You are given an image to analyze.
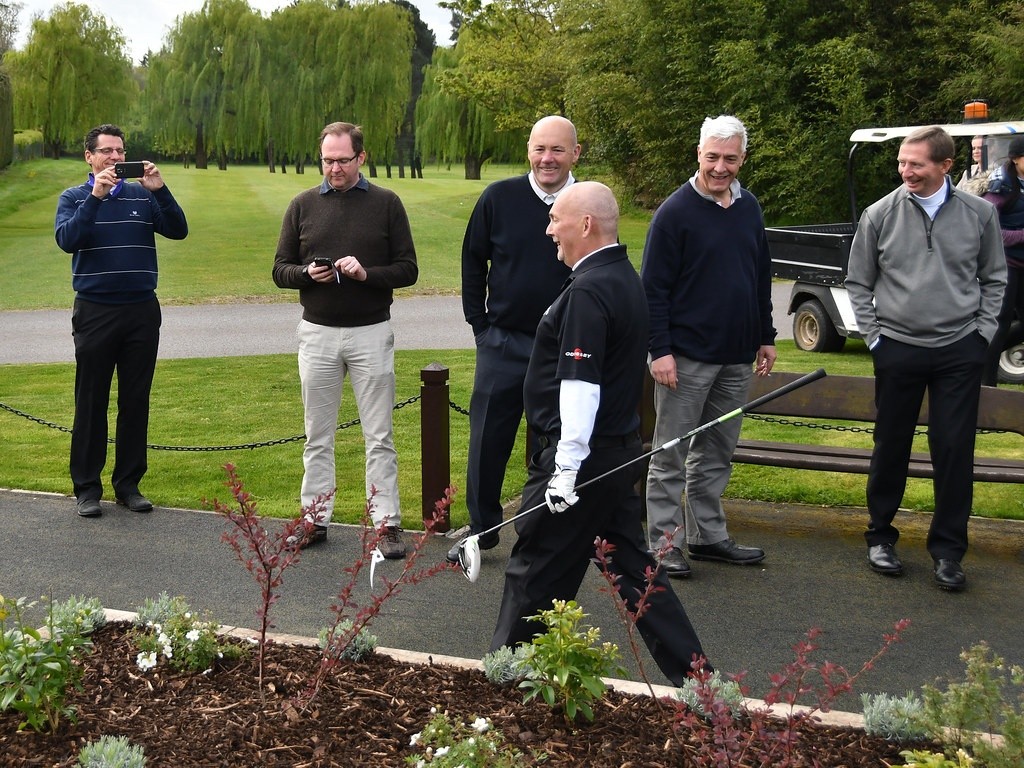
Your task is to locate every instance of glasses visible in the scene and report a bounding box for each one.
[320,152,359,167]
[92,147,127,155]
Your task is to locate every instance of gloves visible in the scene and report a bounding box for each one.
[545,464,579,514]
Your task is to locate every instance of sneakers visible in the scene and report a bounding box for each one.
[284,522,328,550]
[379,525,406,557]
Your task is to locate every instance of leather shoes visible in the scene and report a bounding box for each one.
[687,540,766,564]
[446,529,499,564]
[78,499,102,515]
[655,547,691,575]
[933,559,966,586]
[116,490,153,511]
[868,543,903,572]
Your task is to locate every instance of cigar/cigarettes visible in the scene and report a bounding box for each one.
[767,373,771,376]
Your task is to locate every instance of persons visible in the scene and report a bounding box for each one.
[492,182,719,689]
[846,127,1008,589]
[274,121,419,558]
[981,136,1024,387]
[443,115,620,562]
[955,134,1002,196]
[643,115,779,574]
[55,124,190,516]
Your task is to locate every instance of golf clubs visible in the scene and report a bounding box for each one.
[456,366,828,585]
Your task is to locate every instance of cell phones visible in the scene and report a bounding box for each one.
[314,258,333,273]
[114,162,144,179]
